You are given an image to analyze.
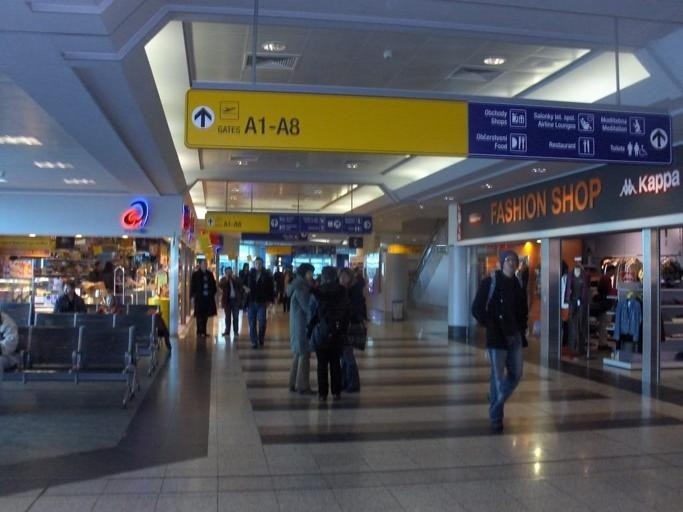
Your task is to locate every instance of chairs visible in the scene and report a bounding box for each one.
[0,303,157,405]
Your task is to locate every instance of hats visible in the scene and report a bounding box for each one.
[499,250,519,267]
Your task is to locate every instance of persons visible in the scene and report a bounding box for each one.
[471,251,529,433]
[54,261,118,372]
[0,312,20,373]
[219,257,368,401]
[190,260,217,337]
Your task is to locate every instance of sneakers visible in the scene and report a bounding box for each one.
[492,421,503,432]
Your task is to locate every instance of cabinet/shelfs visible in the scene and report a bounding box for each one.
[604,284,683,370]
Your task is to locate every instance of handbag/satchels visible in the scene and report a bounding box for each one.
[347,327,366,350]
[240,292,247,308]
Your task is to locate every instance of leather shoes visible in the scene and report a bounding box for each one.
[290,385,359,400]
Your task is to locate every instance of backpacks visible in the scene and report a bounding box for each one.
[312,287,351,342]
